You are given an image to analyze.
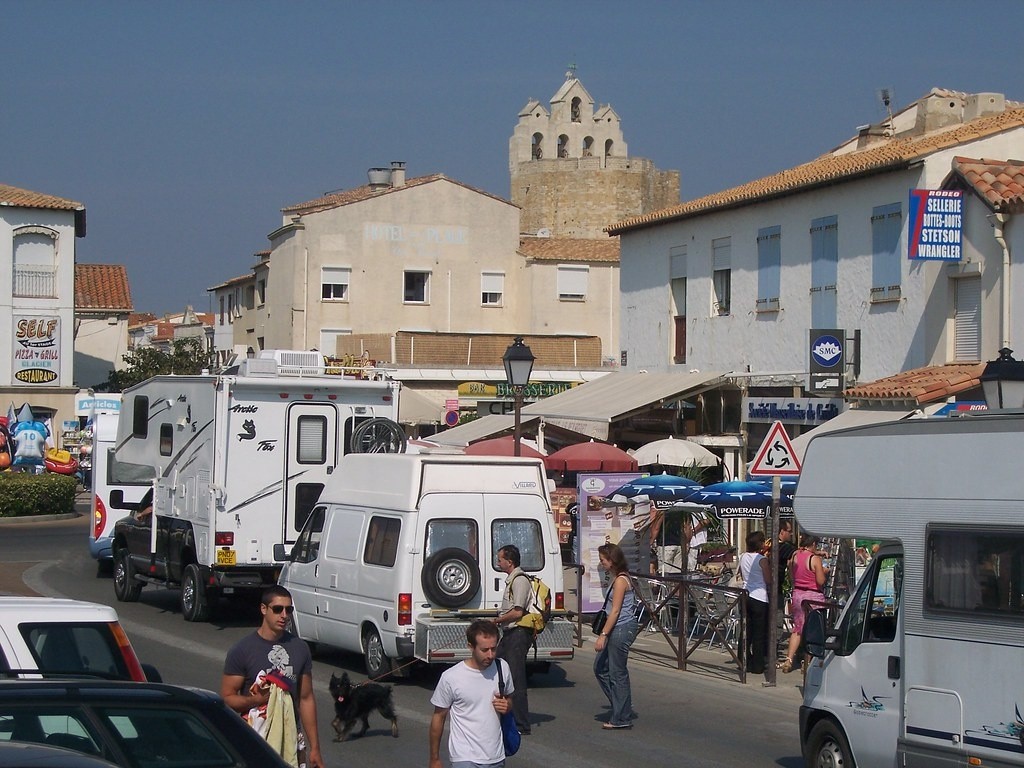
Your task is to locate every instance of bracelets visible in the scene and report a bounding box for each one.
[601,631,609,637]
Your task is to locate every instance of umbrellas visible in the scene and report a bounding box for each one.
[463,435,721,471]
[398,382,458,426]
[604,471,798,627]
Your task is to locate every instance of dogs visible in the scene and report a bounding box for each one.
[328,671,399,741]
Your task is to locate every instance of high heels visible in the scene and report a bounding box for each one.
[784,658,793,673]
[801,660,809,673]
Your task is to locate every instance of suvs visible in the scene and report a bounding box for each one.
[0,670,298,768]
[0,594,164,739]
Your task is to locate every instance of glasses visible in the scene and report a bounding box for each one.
[267,605,294,614]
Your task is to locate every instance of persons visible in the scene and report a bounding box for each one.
[220,586,324,768]
[137,506,152,520]
[649,545,658,575]
[859,544,879,567]
[687,508,711,571]
[79,447,89,473]
[593,544,638,729]
[739,516,832,674]
[493,544,533,735]
[429,620,514,768]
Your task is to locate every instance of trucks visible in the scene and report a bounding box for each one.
[90,413,155,574]
[108,350,400,620]
[788,411,1023,767]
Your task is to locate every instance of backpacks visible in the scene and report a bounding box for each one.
[510,572,552,633]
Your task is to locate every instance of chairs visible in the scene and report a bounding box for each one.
[631,573,798,653]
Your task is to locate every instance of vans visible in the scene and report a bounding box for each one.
[274,454,573,676]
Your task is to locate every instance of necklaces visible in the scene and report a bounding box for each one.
[799,548,814,553]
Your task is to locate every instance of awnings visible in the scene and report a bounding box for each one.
[747,407,919,476]
[422,414,540,446]
[507,370,733,440]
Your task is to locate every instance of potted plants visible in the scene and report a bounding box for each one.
[697,540,735,564]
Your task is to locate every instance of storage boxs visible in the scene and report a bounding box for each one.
[412,618,575,664]
[549,488,577,544]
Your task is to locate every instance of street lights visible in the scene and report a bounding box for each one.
[499,335,535,458]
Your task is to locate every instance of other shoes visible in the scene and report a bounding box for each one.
[602,721,632,730]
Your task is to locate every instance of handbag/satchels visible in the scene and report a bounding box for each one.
[593,610,607,634]
[495,658,522,756]
